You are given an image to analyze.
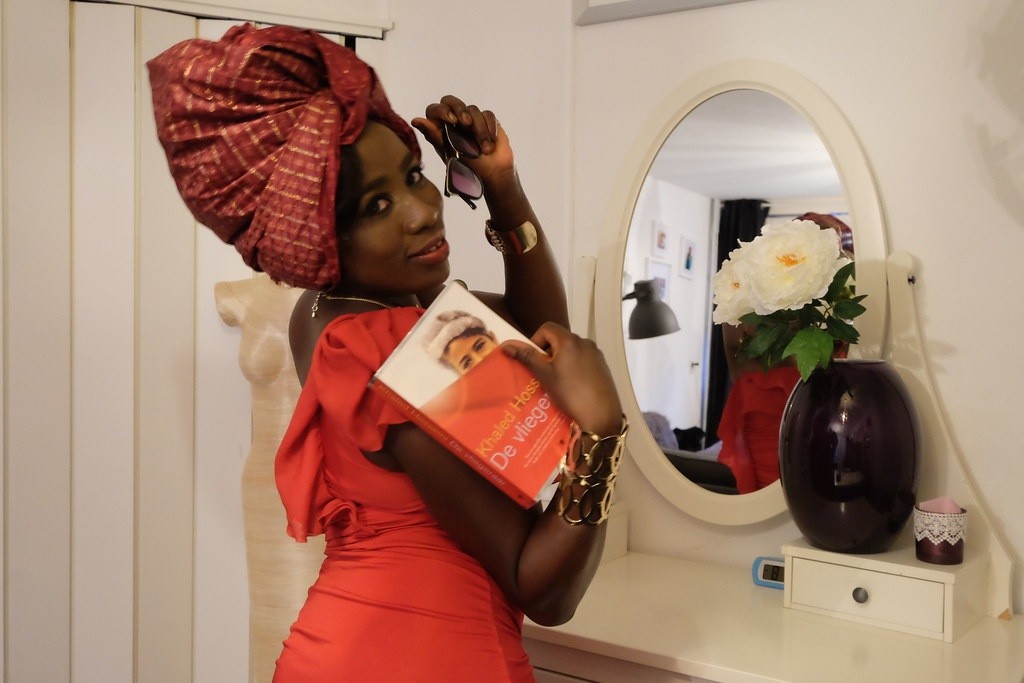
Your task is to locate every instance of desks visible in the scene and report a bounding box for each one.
[523,550,1024,683]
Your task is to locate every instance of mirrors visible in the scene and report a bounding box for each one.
[593,60,887,526]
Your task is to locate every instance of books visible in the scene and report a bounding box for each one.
[368,280,574,509]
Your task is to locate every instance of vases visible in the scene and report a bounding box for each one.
[779,358,915,552]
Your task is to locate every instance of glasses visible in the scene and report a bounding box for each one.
[442,118,484,210]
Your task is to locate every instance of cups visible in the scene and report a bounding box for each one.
[913,506,968,566]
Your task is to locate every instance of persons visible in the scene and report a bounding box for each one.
[421,310,500,374]
[214,273,329,682]
[144,19,631,683]
[715,212,853,495]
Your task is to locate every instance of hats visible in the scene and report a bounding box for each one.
[420,310,485,359]
[146,22,423,294]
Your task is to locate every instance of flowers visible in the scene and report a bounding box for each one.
[737,219,868,384]
[712,249,798,374]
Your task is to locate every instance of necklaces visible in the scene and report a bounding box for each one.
[310,290,421,321]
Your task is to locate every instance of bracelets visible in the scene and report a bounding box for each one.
[485,218,538,256]
[546,411,629,523]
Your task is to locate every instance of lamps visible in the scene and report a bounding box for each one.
[622,279,680,339]
